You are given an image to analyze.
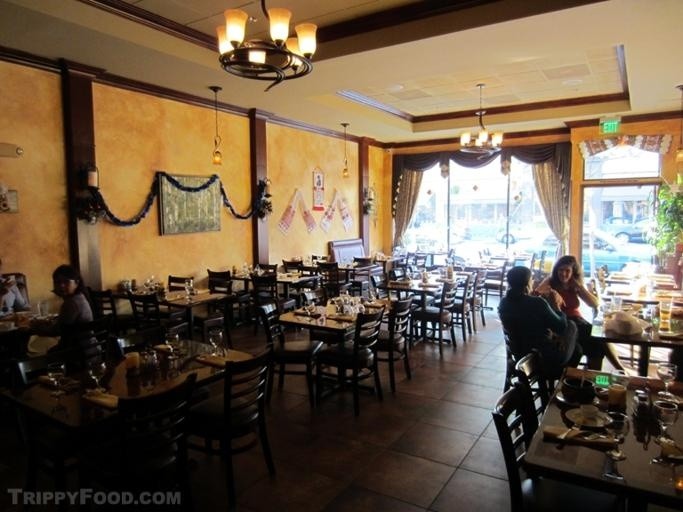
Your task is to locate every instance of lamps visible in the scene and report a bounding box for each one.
[210,86,222,166]
[82,161,106,225]
[215,0,319,94]
[460,84,504,160]
[342,123,348,178]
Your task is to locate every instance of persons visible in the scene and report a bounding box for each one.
[497,265,577,377]
[1,258,32,319]
[14,264,94,397]
[531,255,630,375]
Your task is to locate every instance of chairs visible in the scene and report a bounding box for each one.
[491,380,624,512]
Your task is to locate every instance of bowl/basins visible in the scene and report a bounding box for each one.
[561,377,596,404]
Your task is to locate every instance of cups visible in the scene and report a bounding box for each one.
[659,320,670,333]
[659,299,671,319]
[38,303,48,317]
[610,296,622,310]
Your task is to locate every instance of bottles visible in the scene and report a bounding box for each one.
[149,351,157,385]
[633,390,648,418]
[140,352,149,386]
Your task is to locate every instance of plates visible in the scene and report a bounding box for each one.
[565,408,614,428]
[555,390,600,407]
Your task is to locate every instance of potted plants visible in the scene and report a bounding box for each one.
[654,173,683,288]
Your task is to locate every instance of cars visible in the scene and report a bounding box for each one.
[460,198,658,278]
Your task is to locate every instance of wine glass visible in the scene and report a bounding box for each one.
[185,280,193,301]
[86,360,107,393]
[602,411,630,461]
[47,362,67,400]
[652,401,678,444]
[655,363,678,398]
[611,369,631,386]
[164,328,179,352]
[208,329,223,356]
[304,301,315,321]
